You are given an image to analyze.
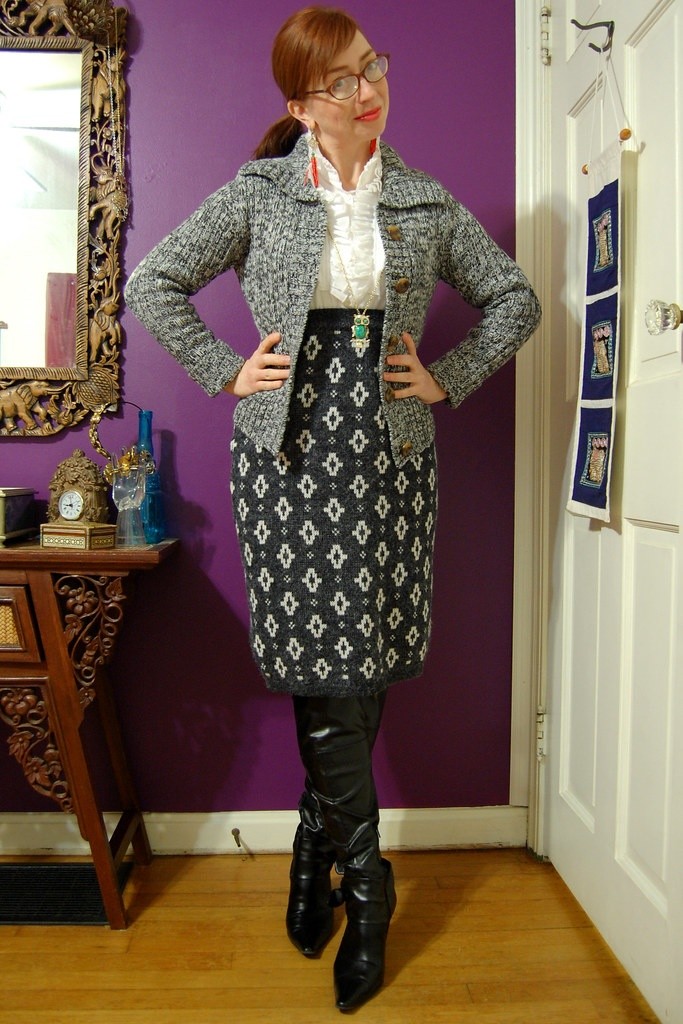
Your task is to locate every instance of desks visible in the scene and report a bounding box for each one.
[0,536,181,930]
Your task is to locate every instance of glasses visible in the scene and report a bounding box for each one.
[303,53,391,101]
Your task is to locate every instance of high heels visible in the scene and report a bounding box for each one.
[333,857,397,1011]
[286,829,334,956]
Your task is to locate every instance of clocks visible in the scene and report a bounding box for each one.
[58,489,85,522]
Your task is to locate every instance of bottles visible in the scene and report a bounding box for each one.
[138,410,165,543]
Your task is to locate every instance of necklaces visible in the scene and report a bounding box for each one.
[327,226,385,359]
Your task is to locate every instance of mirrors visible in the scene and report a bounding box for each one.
[1,0,132,439]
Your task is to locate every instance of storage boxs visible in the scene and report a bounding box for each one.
[39,521,116,551]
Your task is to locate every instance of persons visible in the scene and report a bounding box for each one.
[121,6,543,1013]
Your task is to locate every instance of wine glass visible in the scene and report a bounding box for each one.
[111,454,146,546]
